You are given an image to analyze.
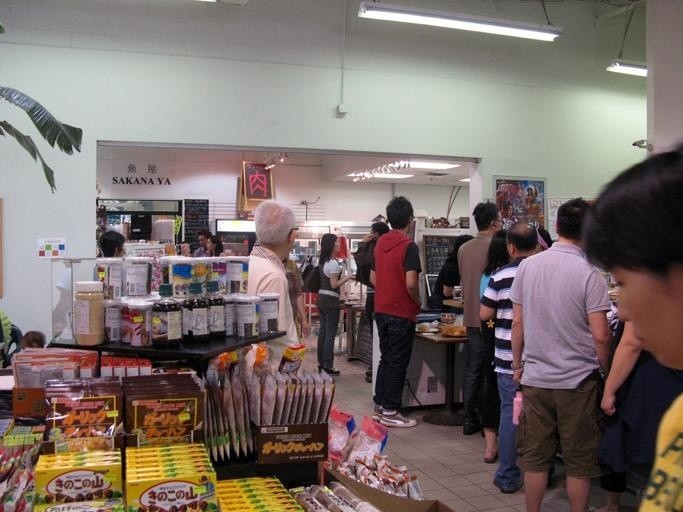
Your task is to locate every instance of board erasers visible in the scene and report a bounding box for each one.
[185,199,208,243]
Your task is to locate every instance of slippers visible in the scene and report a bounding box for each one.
[484,451,498,463]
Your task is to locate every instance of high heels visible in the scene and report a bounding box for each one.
[318,366,340,375]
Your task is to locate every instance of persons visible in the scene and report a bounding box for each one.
[363,196,422,429]
[598,309,664,512]
[251,198,311,369]
[431,193,599,510]
[319,233,357,376]
[583,144,681,512]
[180,230,223,258]
[99,230,125,257]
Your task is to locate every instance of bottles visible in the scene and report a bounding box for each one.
[150,281,227,349]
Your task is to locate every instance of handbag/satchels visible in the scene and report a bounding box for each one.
[301,267,321,293]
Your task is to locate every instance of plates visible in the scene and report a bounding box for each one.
[414,328,465,337]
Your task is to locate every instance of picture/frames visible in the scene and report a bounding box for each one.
[491,174,547,233]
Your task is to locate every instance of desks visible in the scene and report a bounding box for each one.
[415,324,470,427]
[52,328,287,376]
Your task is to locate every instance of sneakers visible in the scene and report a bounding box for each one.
[373,406,383,422]
[380,410,417,428]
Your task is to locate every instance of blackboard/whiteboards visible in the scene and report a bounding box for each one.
[425,273,448,309]
[242,161,273,201]
[422,234,456,295]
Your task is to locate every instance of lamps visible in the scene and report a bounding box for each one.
[357,0,562,43]
[261,151,289,170]
[606,1,647,77]
[351,159,410,183]
